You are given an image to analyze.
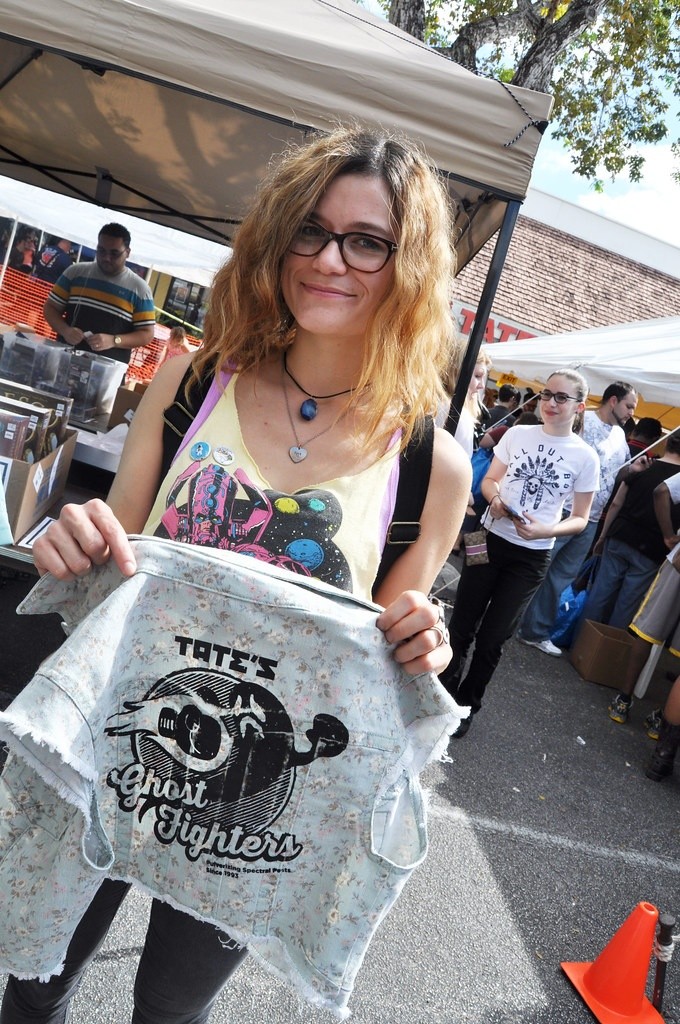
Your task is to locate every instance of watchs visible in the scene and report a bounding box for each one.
[113,335,122,348]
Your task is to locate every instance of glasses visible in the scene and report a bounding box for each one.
[526,404,537,408]
[539,390,580,404]
[96,245,125,258]
[286,217,398,273]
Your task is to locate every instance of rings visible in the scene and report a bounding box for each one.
[430,626,445,647]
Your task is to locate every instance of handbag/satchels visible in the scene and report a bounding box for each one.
[469,446,494,501]
[550,554,602,644]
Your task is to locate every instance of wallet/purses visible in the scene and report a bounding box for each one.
[464,531,489,567]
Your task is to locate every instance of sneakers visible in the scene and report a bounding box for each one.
[644,709,664,739]
[608,694,634,723]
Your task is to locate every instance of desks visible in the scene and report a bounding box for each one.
[65,423,129,472]
[0,484,115,577]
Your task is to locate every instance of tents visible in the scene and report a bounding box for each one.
[0,0,555,436]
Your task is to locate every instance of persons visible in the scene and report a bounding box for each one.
[42,222,156,385]
[153,326,192,374]
[0,125,474,1024]
[582,416,680,782]
[515,380,657,658]
[7,228,73,329]
[434,338,542,557]
[438,369,600,739]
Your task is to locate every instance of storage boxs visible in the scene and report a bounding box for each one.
[108,382,149,430]
[571,618,635,691]
[0,331,129,425]
[0,428,80,546]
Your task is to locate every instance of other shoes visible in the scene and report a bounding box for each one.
[516,628,562,658]
[452,713,473,738]
[451,549,461,556]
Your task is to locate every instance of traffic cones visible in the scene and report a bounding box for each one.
[561,901,666,1024]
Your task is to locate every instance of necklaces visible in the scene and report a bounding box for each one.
[282,352,375,464]
[284,349,375,422]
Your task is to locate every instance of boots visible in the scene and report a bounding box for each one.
[646,720,680,782]
